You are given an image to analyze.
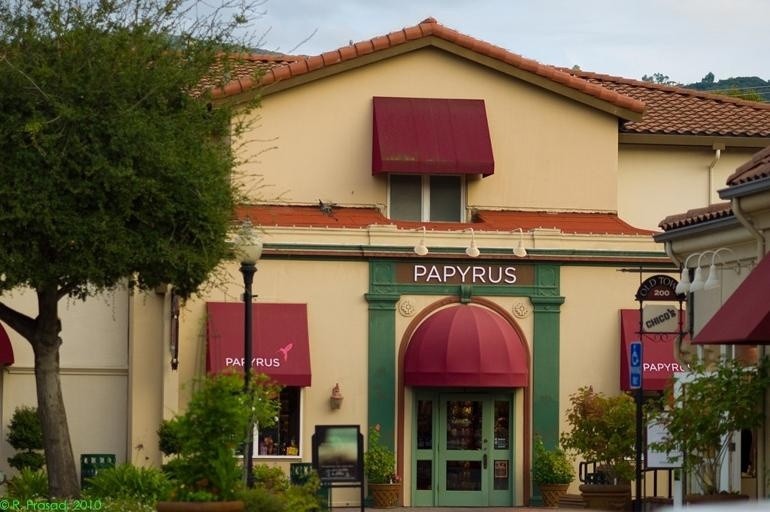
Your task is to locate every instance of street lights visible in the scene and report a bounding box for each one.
[233,216,264,492]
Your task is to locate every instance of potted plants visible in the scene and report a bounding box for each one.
[531,381,638,511]
[151,365,283,511]
[363,424,403,509]
[641,345,770,511]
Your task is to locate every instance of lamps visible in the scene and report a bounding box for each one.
[673,247,743,294]
[330,382,344,410]
[414,224,528,261]
